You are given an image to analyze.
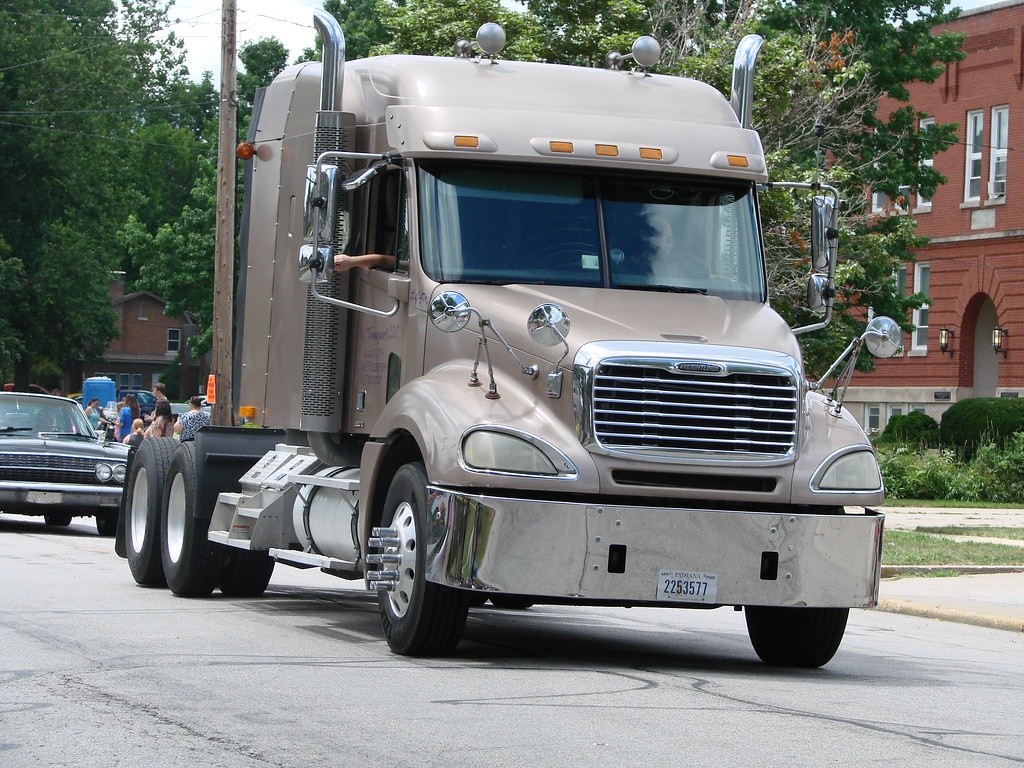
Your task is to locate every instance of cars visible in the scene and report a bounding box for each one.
[74,390,159,423]
[167,395,212,423]
[0,391,132,540]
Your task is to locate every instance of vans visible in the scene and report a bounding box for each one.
[3,383,51,395]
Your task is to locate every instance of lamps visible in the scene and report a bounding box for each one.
[992,322,1008,358]
[938,325,954,357]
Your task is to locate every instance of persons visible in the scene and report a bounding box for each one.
[628,213,735,282]
[85,398,111,430]
[39,389,70,432]
[173,397,211,442]
[332,254,409,274]
[111,394,140,443]
[122,418,144,447]
[145,382,174,438]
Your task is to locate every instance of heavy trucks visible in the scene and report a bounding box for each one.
[113,0,902,669]
